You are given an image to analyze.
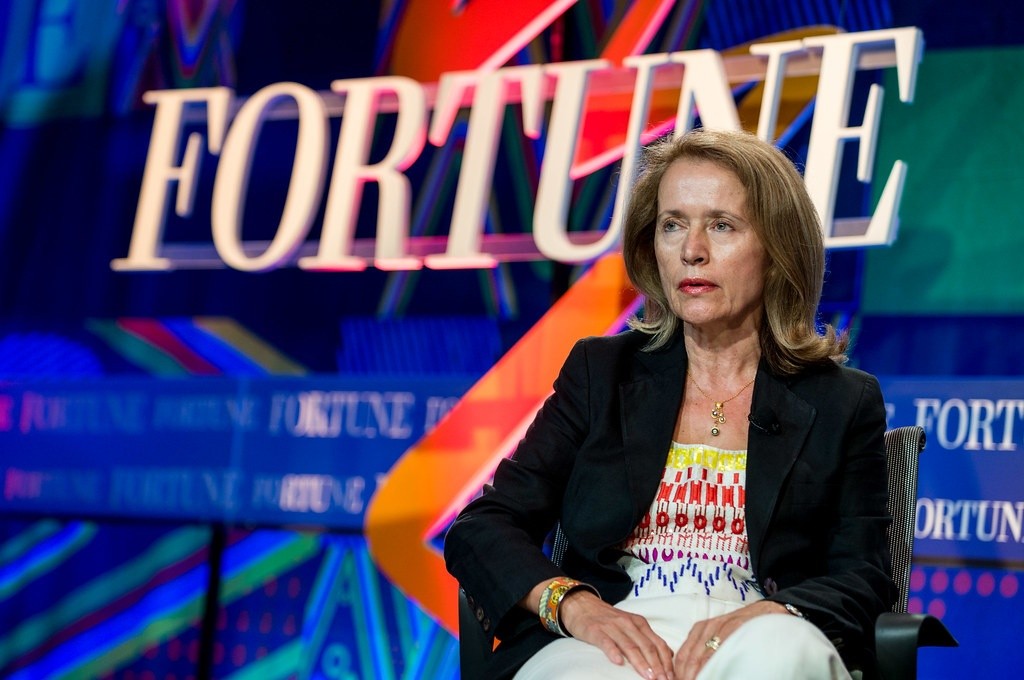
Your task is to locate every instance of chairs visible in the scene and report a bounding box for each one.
[456,426,959,680]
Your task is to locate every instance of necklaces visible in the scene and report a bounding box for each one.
[686,371,755,436]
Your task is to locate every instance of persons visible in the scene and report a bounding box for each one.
[444,130,900,680]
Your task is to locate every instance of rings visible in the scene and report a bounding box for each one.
[705,635,721,650]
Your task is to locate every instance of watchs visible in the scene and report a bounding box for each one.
[785,603,804,617]
